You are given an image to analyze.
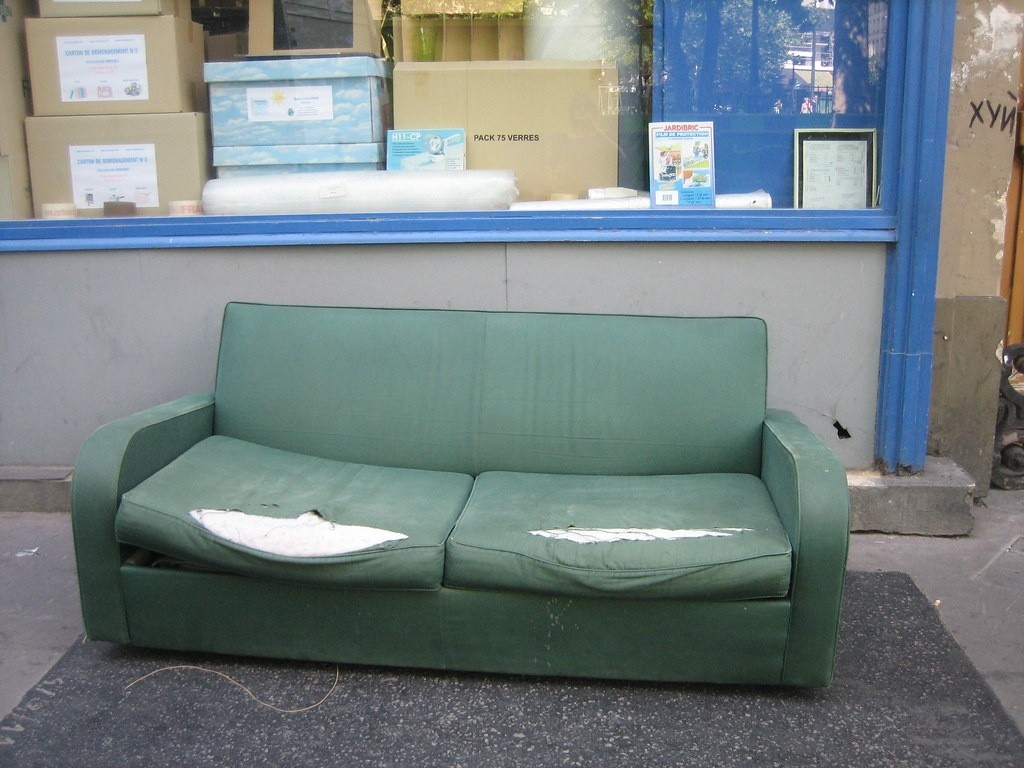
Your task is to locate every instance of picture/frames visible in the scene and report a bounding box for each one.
[793,128,877,210]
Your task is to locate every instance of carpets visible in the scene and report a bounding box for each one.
[0,580,1024,768]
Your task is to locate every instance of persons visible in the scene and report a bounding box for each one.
[774,99,782,114]
[809,95,817,116]
[800,97,813,114]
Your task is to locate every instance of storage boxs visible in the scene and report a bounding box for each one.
[0,0,619,221]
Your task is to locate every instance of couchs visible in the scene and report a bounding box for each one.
[72,300,852,692]
